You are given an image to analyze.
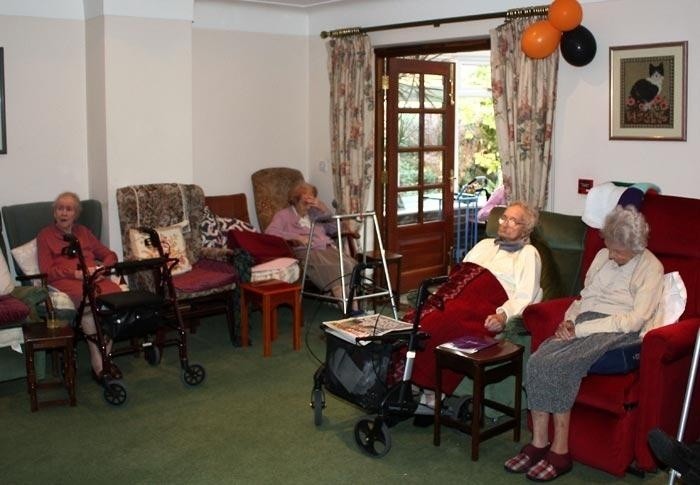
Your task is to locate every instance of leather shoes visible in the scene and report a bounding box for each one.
[104,361,122,379]
[90,366,112,383]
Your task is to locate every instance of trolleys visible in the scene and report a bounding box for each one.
[64,227,206,407]
[309,262,485,460]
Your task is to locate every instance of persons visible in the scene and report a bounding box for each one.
[264,183,358,317]
[505,204,664,481]
[386,201,541,400]
[37,192,123,382]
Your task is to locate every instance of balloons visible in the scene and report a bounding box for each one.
[522,20,560,58]
[549,0,582,31]
[560,24,596,67]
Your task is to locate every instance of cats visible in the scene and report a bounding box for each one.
[630,61,665,110]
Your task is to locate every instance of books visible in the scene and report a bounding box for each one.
[440,336,500,353]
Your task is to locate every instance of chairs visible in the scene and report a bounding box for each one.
[116,182,254,347]
[451,207,586,421]
[252,166,359,259]
[522,191,699,476]
[0,199,143,399]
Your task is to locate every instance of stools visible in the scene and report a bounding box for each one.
[436,340,524,460]
[25,323,76,414]
[241,279,301,358]
[354,249,403,312]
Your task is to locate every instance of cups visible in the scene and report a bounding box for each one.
[46,310,59,331]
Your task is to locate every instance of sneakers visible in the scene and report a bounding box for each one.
[413,394,436,427]
[505,443,551,472]
[526,451,572,481]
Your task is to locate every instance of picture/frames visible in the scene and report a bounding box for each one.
[608,41,688,141]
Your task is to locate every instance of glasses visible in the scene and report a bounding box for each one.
[498,216,525,226]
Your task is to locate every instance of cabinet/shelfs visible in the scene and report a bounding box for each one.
[299,210,402,321]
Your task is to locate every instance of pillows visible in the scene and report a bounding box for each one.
[0,249,15,295]
[216,216,257,233]
[11,238,41,276]
[198,201,224,249]
[126,222,193,276]
